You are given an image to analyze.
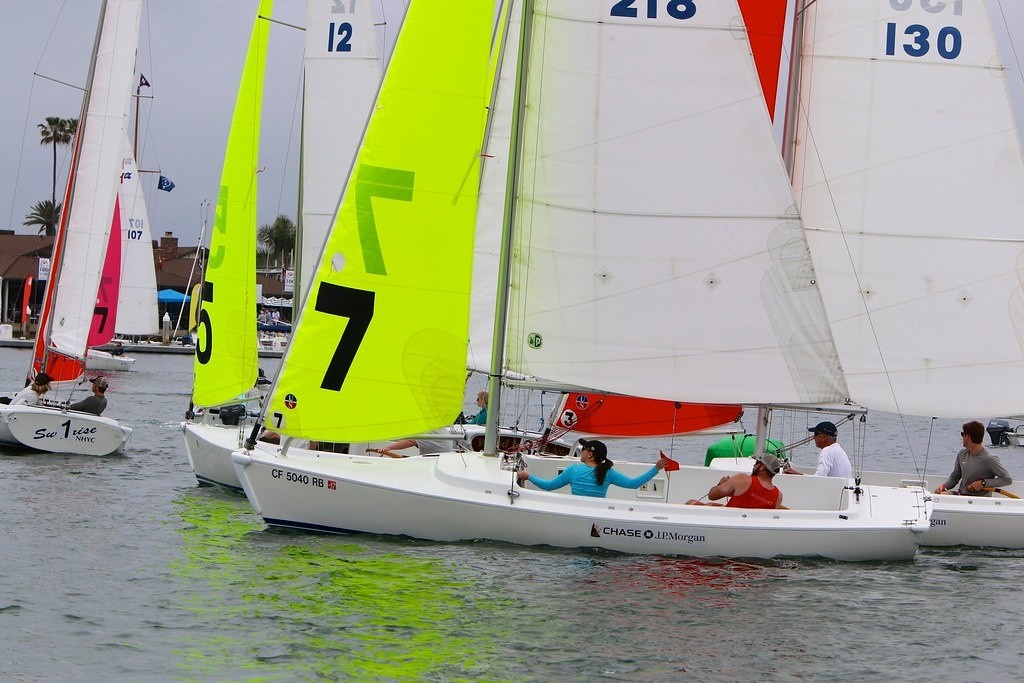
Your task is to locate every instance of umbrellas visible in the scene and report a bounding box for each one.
[157,288,192,313]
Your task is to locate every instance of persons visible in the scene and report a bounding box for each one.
[68,375,110,415]
[377,427,454,456]
[934,420,1012,497]
[784,422,853,478]
[258,308,280,323]
[465,392,489,424]
[708,454,782,509]
[517,438,668,499]
[240,368,273,412]
[263,430,317,450]
[8,373,54,406]
[110,342,125,357]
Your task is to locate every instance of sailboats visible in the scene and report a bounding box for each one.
[180,0,1024,562]
[0,1,175,458]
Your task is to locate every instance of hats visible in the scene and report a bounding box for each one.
[89,376,109,388]
[809,421,838,437]
[579,437,607,459]
[35,373,53,386]
[753,454,780,474]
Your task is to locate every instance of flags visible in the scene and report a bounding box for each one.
[140,75,150,87]
[158,176,176,191]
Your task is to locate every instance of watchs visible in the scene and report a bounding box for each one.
[982,479,986,487]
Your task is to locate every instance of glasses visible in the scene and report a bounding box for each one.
[814,432,824,436]
[961,432,967,436]
[117,345,121,347]
[582,446,591,452]
[477,398,480,401]
[756,460,763,465]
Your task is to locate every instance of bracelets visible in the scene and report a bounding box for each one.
[940,484,944,487]
